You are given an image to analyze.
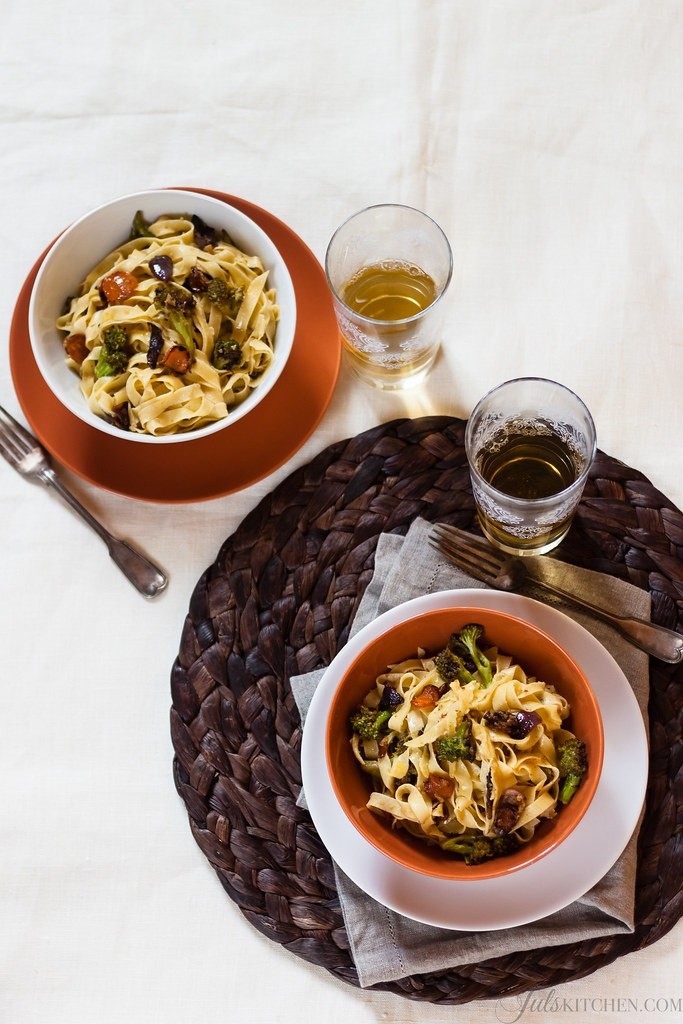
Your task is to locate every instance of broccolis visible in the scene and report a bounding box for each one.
[350,624,585,866]
[93,210,244,382]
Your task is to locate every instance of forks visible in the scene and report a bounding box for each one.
[0,406,170,600]
[427,522,683,666]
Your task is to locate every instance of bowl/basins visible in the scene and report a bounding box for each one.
[28,189,297,445]
[324,608,605,881]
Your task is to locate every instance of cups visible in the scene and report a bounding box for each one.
[464,377,597,557]
[326,204,453,393]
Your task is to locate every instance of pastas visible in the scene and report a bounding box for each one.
[56,216,280,436]
[350,648,585,863]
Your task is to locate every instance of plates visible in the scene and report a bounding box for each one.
[300,588,650,932]
[8,186,340,506]
